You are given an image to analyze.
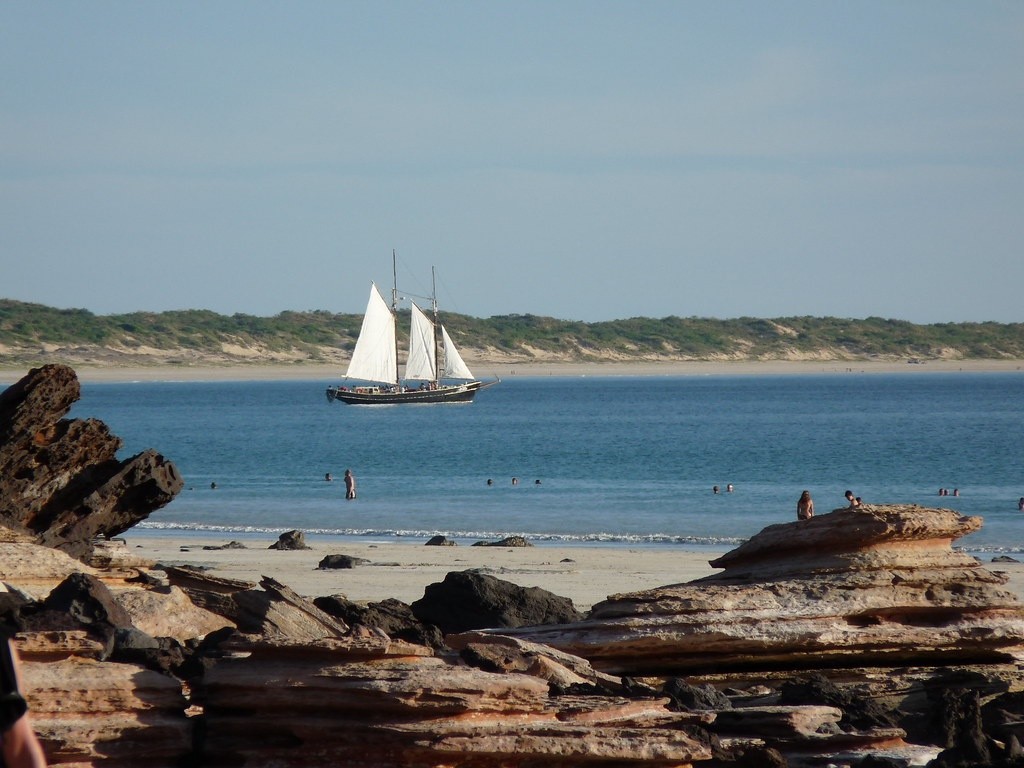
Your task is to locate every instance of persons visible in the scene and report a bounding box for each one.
[938,488,959,497]
[797,490,814,520]
[845,490,863,508]
[344,469,358,500]
[535,480,541,484]
[211,482,216,489]
[727,483,733,493]
[511,478,517,485]
[713,486,719,494]
[325,473,331,481]
[329,381,447,394]
[1018,497,1024,511]
[487,479,493,486]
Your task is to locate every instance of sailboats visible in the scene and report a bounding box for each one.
[325,248,502,405]
